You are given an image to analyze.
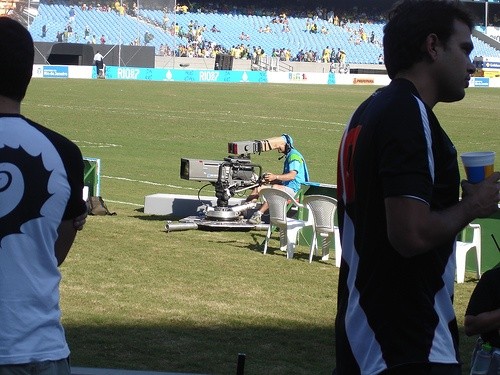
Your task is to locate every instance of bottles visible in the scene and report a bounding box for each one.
[470,343,492,375]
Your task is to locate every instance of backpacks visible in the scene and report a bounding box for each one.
[87,196,117,216]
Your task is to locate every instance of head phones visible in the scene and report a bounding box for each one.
[282,134,291,153]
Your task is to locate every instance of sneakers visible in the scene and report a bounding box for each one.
[241,202,256,209]
[249,212,261,224]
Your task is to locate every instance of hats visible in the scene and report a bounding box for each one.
[282,134,293,147]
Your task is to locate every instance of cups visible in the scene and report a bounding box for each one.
[459,151,496,187]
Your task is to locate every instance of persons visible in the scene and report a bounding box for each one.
[0,17,87,375]
[42,0,385,77]
[334,0,500,375]
[240,133,309,224]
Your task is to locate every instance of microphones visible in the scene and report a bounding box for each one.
[278,151,289,160]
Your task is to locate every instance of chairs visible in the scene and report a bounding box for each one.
[303,194,346,268]
[29,4,500,64]
[259,187,319,258]
[452,224,482,285]
